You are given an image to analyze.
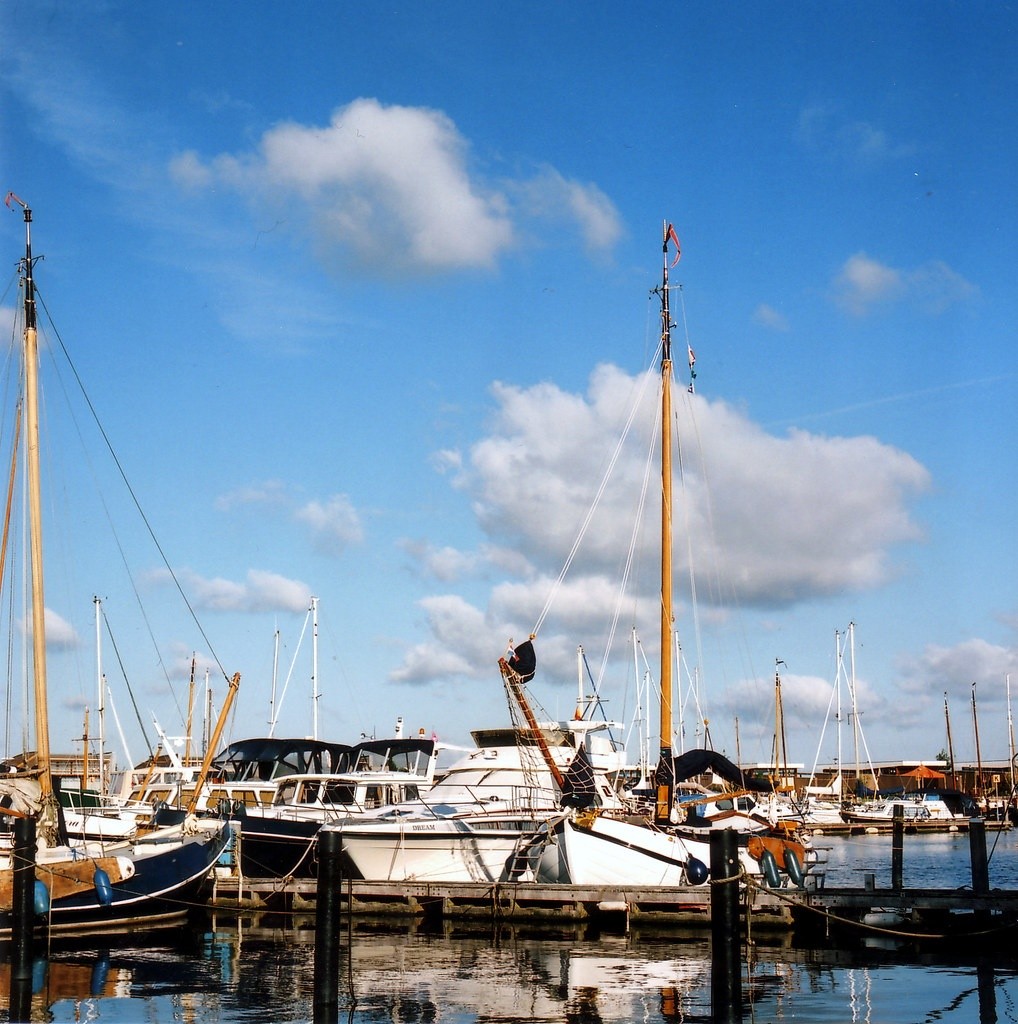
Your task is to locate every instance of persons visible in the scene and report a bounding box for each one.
[921,805,931,822]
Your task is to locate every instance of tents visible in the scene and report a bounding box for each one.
[878,765,947,792]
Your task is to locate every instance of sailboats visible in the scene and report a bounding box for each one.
[0,190,1018,946]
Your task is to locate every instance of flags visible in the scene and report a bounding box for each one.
[431,730,439,743]
[574,707,581,721]
[507,644,519,662]
[686,344,697,394]
